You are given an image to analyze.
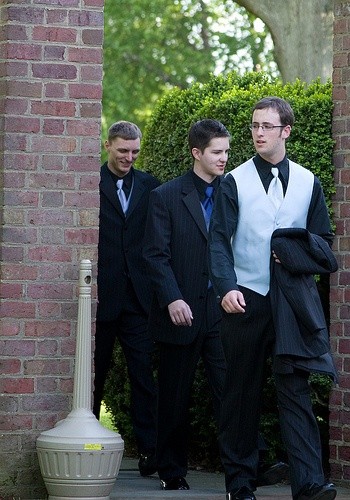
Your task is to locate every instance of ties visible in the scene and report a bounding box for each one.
[266,166,285,198]
[201,187,212,226]
[116,176,128,211]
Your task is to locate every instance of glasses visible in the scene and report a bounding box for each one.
[247,121,287,133]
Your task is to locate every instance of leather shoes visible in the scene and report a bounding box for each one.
[160,476,188,491]
[250,461,289,492]
[291,483,337,500]
[226,486,256,500]
[139,455,157,476]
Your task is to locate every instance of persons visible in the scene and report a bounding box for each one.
[93,120,163,477]
[208,96,339,500]
[143,119,292,491]
